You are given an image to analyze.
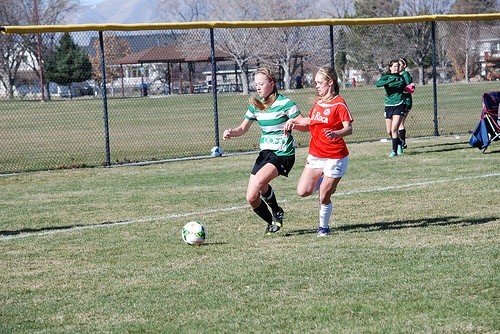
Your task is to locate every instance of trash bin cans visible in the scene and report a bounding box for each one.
[140,83,148,97]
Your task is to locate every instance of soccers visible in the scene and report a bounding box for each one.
[181,221,206,246]
[210,146,223,157]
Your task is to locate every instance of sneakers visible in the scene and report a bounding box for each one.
[317,227,329,237]
[398,145,403,155]
[402,144,407,149]
[388,151,397,157]
[270,207,284,231]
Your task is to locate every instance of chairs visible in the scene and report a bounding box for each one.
[482,91,500,153]
